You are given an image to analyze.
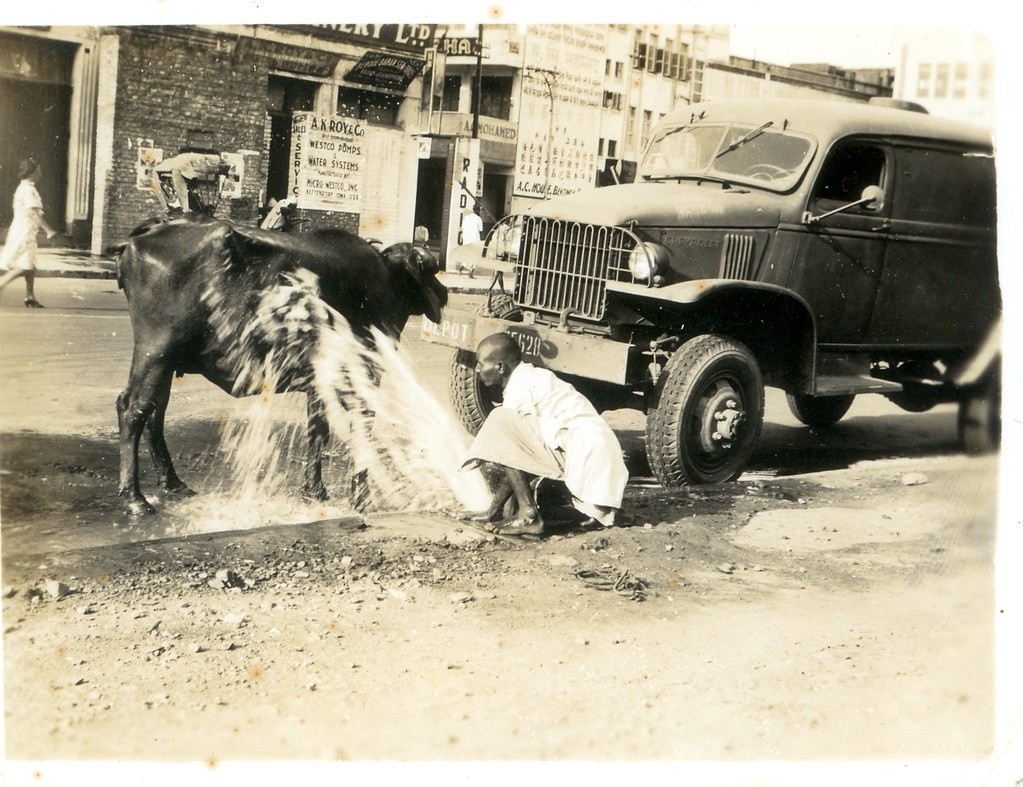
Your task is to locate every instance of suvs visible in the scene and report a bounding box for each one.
[419,94,1001,493]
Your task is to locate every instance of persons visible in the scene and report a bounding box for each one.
[0,158,57,308]
[454,333,626,534]
[457,204,483,279]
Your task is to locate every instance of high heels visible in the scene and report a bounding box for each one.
[23,296,44,308]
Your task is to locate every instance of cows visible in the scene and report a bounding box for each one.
[115,217,449,519]
